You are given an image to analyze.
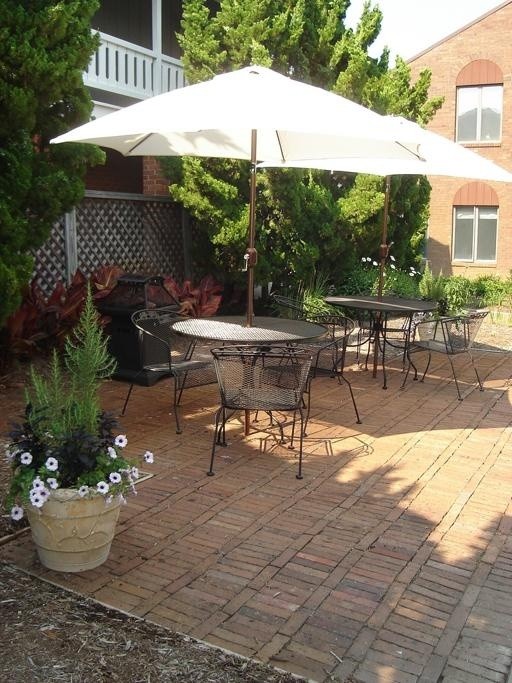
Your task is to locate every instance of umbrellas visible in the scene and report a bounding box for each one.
[48,64,432,435]
[254,116,512,378]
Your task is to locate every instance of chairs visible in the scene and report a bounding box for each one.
[121,309,225,435]
[400,311,490,401]
[206,345,318,479]
[273,295,346,379]
[253,314,362,437]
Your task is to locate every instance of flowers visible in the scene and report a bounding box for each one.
[4,281,153,521]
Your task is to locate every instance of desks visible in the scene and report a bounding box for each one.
[325,295,436,390]
[169,316,329,447]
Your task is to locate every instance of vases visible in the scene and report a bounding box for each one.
[28,488,121,573]
